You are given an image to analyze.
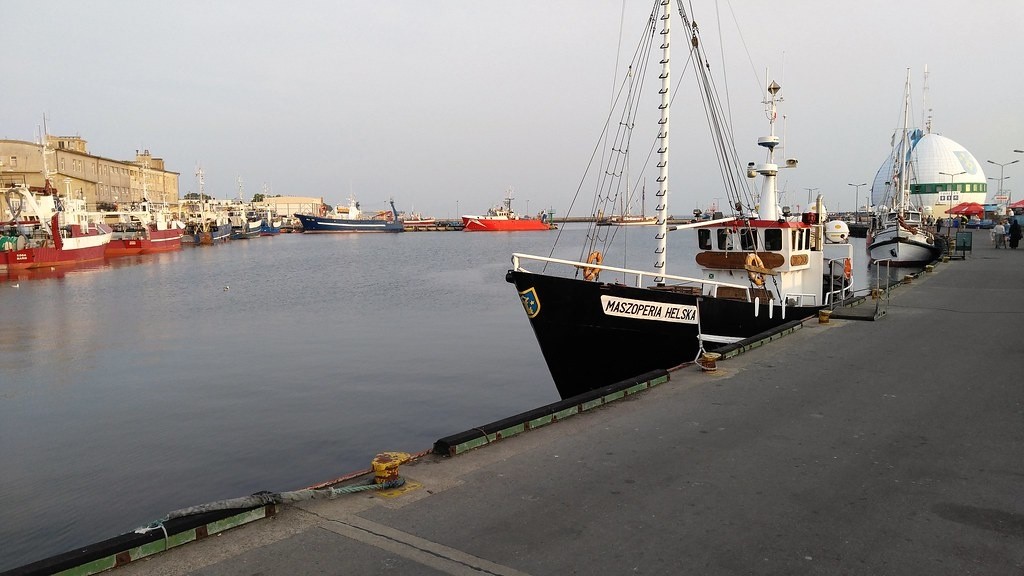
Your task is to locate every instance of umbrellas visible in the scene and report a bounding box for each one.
[945,202,983,214]
[1007,200,1024,208]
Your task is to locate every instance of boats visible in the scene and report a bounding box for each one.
[461,185,551,231]
[227,177,263,240]
[259,183,282,236]
[102,159,187,255]
[503,0,854,398]
[0,113,114,271]
[177,167,233,244]
[867,72,937,262]
[292,190,405,233]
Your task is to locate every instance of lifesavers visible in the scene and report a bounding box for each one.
[845,259,851,280]
[745,253,767,285]
[583,251,602,280]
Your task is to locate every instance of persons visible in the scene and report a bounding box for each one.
[927,215,943,232]
[954,214,968,232]
[992,220,1022,249]
[974,217,981,231]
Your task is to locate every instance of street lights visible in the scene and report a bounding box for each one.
[987,177,1010,196]
[939,171,967,236]
[802,187,819,202]
[848,183,868,225]
[988,160,1020,203]
